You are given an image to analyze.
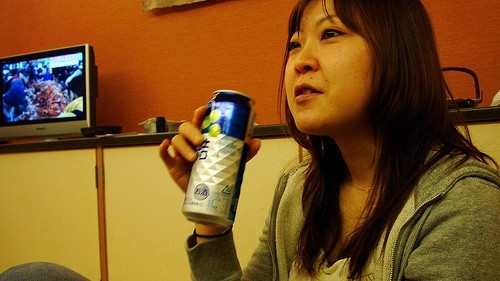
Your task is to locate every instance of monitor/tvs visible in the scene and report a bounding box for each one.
[0,44,96,140]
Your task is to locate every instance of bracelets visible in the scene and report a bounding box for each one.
[191,224,235,240]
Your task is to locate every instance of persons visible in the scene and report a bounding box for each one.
[56,69,84,118]
[157,2,500,281]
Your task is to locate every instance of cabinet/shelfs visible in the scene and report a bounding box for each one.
[0,103,500,281]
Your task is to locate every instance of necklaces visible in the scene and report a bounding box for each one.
[347,180,375,196]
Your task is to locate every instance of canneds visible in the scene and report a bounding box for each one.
[182,90,256,226]
